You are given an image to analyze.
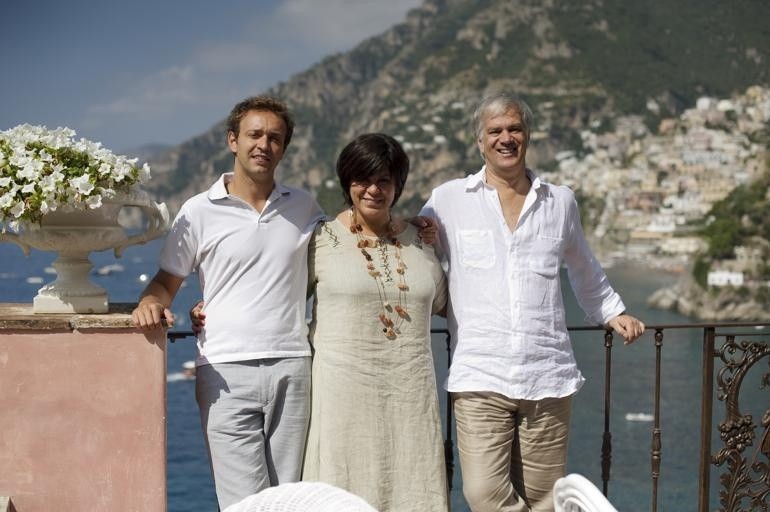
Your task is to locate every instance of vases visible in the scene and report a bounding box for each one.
[0,189,170,314]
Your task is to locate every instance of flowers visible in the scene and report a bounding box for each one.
[0,122,152,234]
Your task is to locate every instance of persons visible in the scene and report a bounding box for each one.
[131,95,445,512]
[189,132,452,512]
[418,93,647,512]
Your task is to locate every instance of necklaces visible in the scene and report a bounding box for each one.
[349,204,411,339]
